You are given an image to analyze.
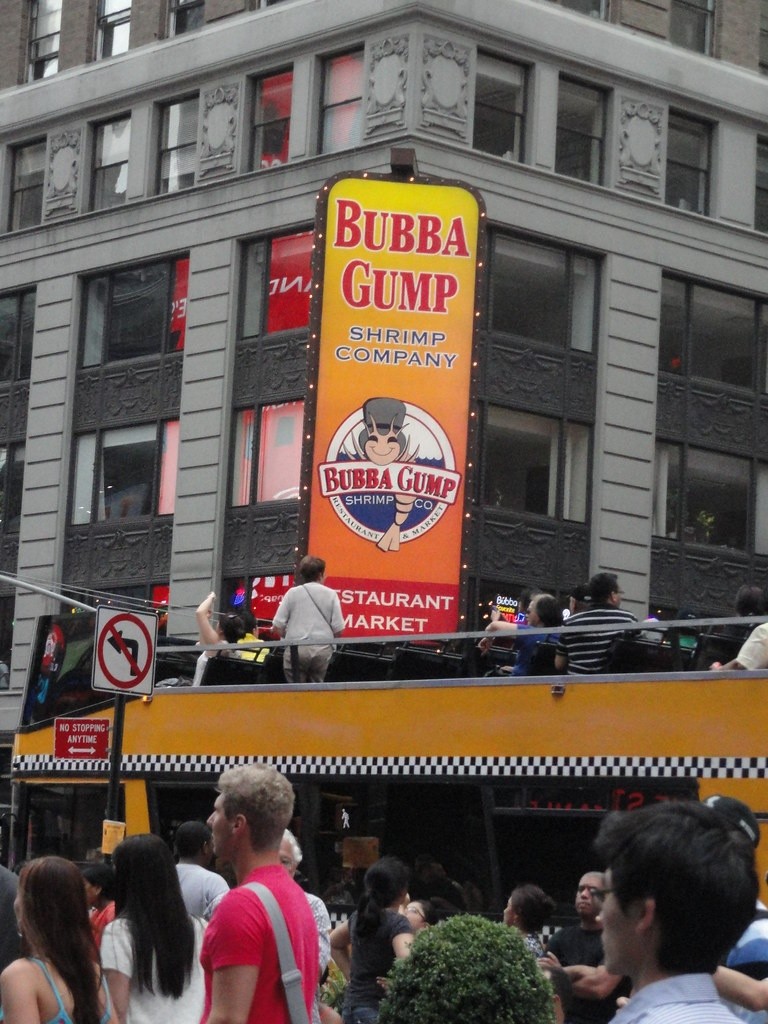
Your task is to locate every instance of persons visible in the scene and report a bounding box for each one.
[194,591,271,688]
[273,555,346,684]
[0,654,9,688]
[478,571,768,677]
[0,760,768,1024]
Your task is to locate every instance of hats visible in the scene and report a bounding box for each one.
[703,794,760,848]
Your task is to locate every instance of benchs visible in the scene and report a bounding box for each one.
[324,640,408,682]
[200,647,273,685]
[460,636,518,677]
[687,614,756,671]
[154,636,202,684]
[602,627,700,673]
[529,633,568,676]
[385,640,462,680]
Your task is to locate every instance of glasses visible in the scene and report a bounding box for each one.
[617,590,625,594]
[591,887,614,901]
[526,610,530,614]
[228,614,238,620]
